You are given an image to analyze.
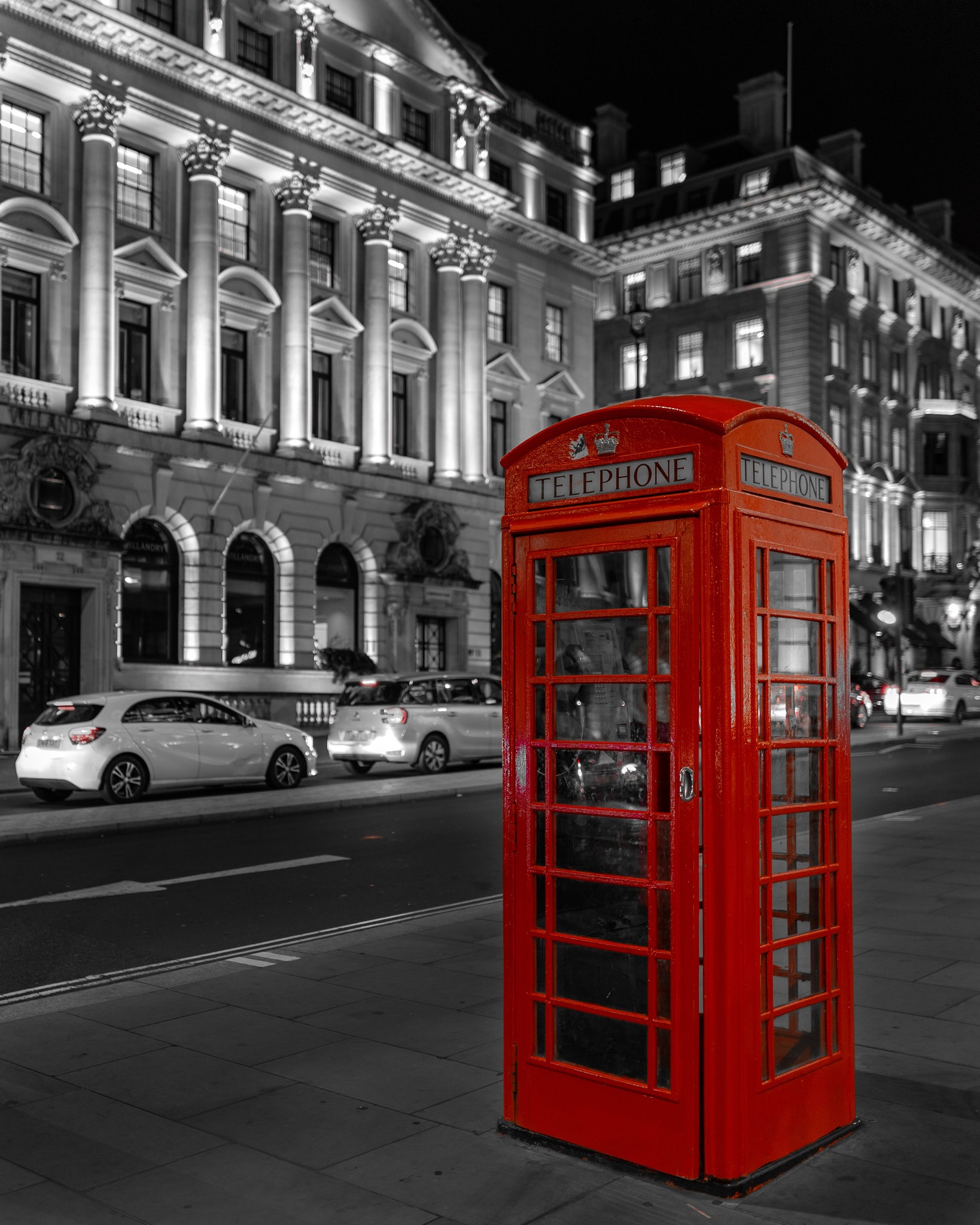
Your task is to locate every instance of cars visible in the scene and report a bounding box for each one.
[15,691,318,804]
[327,672,583,775]
[768,671,892,730]
[883,668,980,726]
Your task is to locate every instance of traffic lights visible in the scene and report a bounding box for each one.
[876,575,902,629]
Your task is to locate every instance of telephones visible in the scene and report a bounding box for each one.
[556,620,629,743]
